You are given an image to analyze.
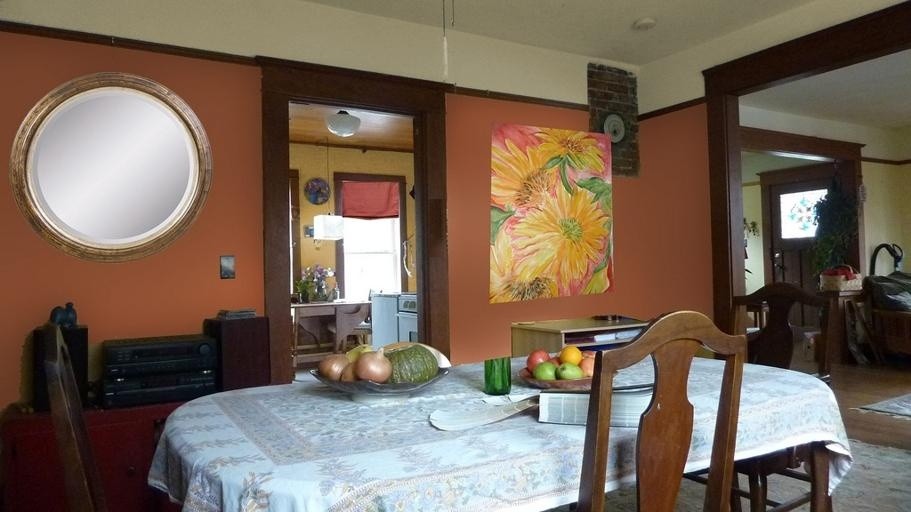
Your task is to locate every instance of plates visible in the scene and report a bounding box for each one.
[519,367,620,390]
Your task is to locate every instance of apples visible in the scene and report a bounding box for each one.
[581,359,595,379]
[581,351,595,357]
[550,356,559,365]
[525,350,553,376]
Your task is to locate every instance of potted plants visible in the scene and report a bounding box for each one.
[295,279,317,303]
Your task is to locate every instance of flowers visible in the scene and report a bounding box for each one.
[303,264,334,288]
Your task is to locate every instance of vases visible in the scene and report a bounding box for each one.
[316,286,326,297]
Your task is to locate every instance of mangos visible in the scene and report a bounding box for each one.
[557,364,583,381]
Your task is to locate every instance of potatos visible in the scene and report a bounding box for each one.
[340,362,354,381]
[345,344,369,361]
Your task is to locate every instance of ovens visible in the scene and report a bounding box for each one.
[399,311,418,343]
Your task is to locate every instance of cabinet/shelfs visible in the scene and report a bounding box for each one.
[510,311,653,359]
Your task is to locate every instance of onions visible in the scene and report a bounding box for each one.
[357,347,392,382]
[318,353,348,380]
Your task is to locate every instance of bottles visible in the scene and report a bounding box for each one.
[332,288,340,301]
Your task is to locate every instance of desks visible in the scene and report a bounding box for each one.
[292,299,373,368]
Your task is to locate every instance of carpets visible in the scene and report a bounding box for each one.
[860,392,911,417]
[554,437,910,512]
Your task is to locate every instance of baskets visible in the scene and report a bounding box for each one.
[820,264,862,293]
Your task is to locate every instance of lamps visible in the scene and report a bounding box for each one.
[313,138,345,241]
[323,107,360,137]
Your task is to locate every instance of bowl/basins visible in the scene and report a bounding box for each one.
[309,341,450,402]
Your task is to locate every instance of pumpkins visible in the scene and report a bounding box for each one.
[383,344,439,383]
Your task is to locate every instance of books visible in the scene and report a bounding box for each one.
[615,329,641,341]
[536,382,654,426]
[564,332,615,345]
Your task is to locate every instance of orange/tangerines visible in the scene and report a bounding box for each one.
[532,363,557,380]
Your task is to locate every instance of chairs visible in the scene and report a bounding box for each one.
[326,289,382,354]
[861,271,911,364]
[40,323,108,511]
[727,283,842,512]
[574,309,749,512]
